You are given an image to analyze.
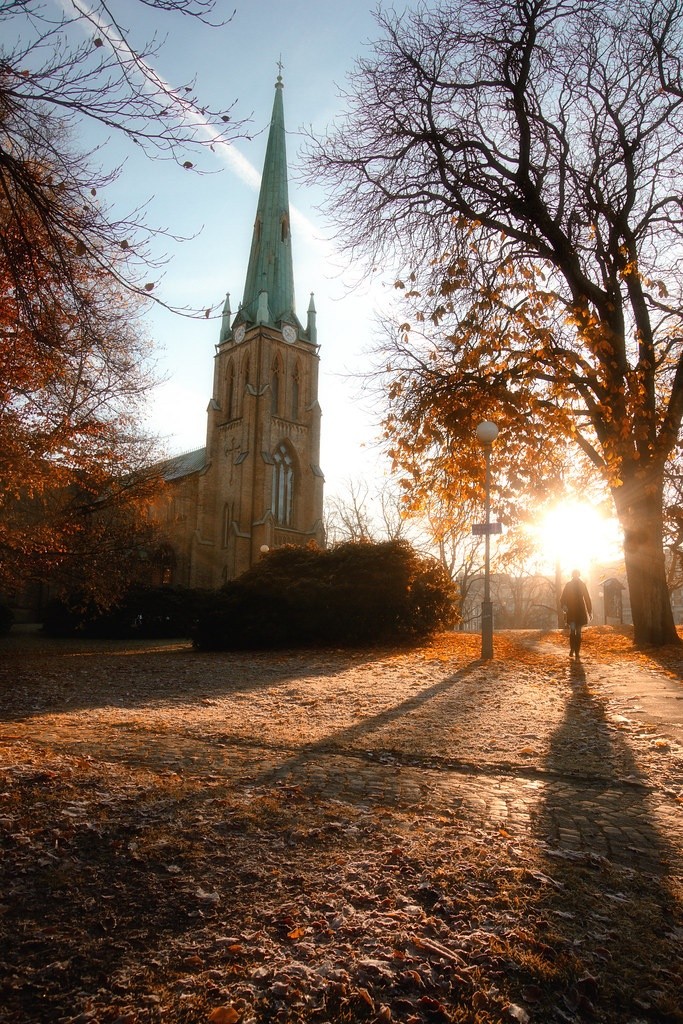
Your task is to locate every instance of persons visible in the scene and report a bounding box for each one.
[560,569,592,659]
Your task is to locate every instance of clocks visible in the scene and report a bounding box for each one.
[234,326,245,344]
[282,326,296,343]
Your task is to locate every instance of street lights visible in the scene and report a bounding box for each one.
[475,422,500,660]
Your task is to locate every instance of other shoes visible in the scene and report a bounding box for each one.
[576,651,579,658]
[569,650,573,656]
